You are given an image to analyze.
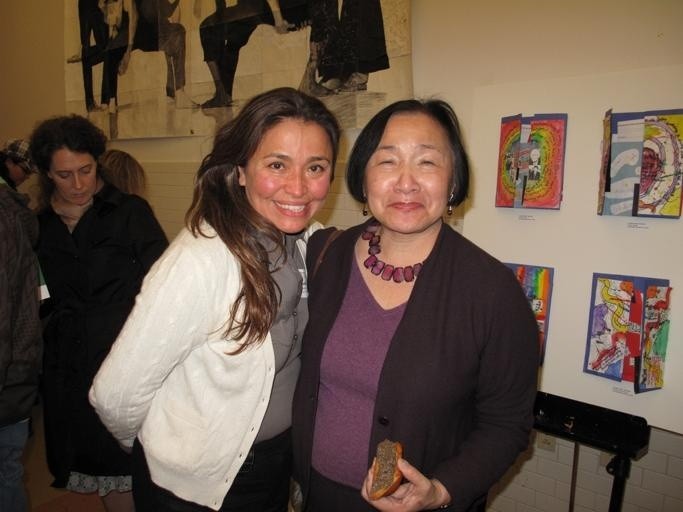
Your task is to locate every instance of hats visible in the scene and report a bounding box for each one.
[2,140,33,169]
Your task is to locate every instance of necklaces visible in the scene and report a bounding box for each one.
[362,218,428,283]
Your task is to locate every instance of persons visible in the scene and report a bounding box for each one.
[292,95,538,510]
[76,0,390,114]
[90,88,345,510]
[1,114,169,511]
